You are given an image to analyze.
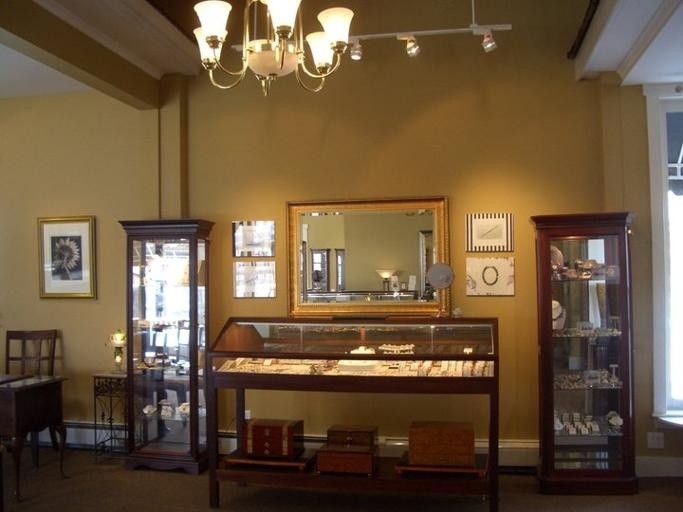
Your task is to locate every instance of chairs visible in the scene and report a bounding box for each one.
[2,328,63,469]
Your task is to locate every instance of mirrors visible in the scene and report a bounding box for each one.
[284,195,455,318]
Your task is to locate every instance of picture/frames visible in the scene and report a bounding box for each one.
[36,216,96,299]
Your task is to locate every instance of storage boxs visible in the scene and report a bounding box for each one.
[313,446,378,474]
[406,418,475,465]
[323,424,379,446]
[241,416,306,458]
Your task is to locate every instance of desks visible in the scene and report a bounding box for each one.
[91,372,130,456]
[0,373,67,502]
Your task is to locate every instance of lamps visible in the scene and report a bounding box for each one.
[103,327,124,373]
[376,268,397,291]
[229,1,512,63]
[192,1,354,99]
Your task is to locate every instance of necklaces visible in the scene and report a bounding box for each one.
[446,357,488,377]
[552,307,564,321]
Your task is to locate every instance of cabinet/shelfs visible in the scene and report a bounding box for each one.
[211,316,501,511]
[530,210,642,497]
[116,219,215,475]
